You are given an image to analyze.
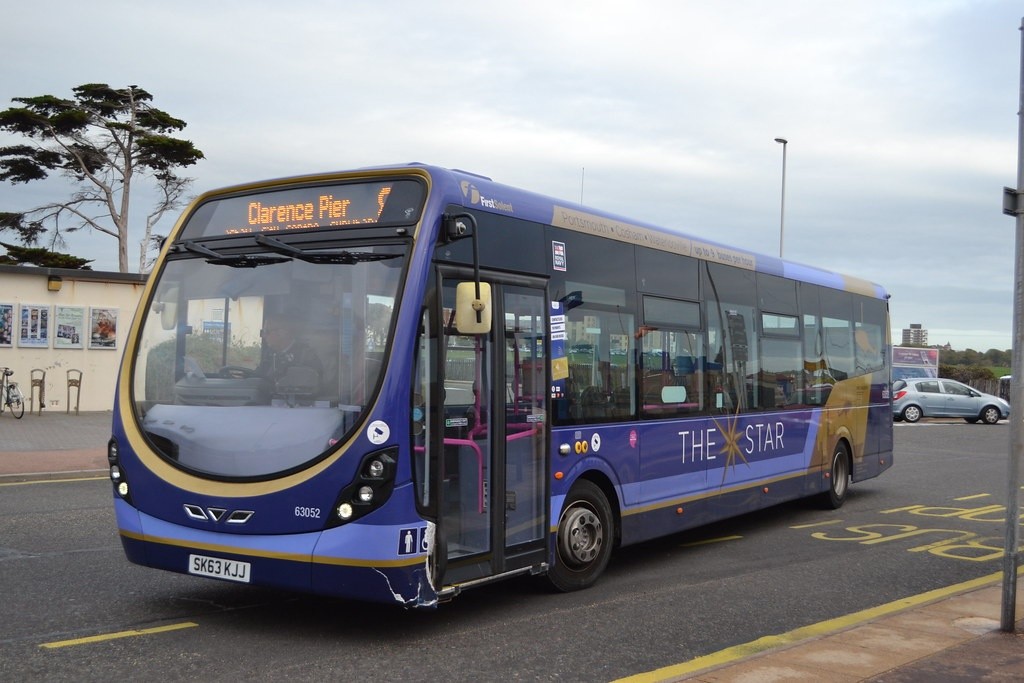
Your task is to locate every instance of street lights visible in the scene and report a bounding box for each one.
[774,137,789,257]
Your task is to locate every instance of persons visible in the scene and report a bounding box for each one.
[252,303,322,404]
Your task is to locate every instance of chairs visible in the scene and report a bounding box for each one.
[582,365,694,422]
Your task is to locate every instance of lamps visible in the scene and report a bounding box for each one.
[48,276,64,291]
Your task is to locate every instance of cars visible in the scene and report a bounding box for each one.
[891,377,1010,424]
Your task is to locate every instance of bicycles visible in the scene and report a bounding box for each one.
[0,366,25,419]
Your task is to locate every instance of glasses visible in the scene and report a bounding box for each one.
[260,328,271,338]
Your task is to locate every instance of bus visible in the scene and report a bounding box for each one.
[105,159,895,617]
